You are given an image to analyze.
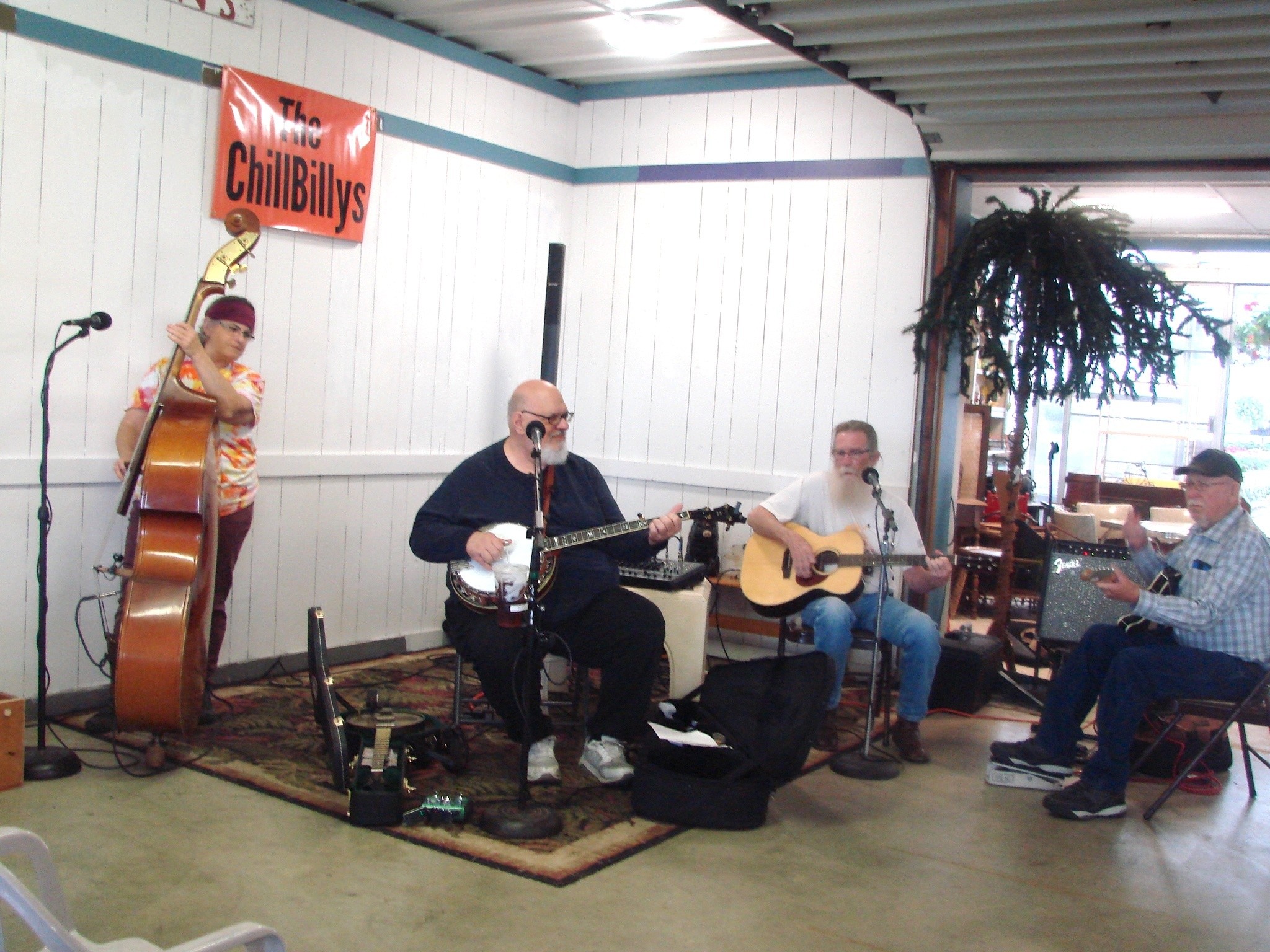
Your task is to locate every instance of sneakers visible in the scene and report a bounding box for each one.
[1043,780,1128,820]
[527,735,560,782]
[580,734,635,785]
[990,735,1075,777]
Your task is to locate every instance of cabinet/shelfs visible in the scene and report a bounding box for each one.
[964,280,1017,455]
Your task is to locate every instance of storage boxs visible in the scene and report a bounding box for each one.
[0,689,28,794]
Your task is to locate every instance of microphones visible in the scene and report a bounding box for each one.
[62,312,113,331]
[526,420,546,455]
[862,466,882,493]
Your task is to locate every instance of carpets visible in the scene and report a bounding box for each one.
[51,643,940,891]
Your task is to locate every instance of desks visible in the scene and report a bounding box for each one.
[948,545,1057,699]
[1098,519,1192,557]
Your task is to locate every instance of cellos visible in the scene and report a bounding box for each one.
[110,208,266,770]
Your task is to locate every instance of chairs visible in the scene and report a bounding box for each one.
[1148,506,1197,546]
[0,824,288,952]
[1128,669,1270,824]
[774,608,893,751]
[1051,505,1099,548]
[1075,500,1135,546]
[434,582,588,799]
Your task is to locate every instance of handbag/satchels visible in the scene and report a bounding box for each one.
[631,652,836,830]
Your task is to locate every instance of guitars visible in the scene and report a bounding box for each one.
[738,523,999,618]
[345,706,427,784]
[446,502,748,613]
[1079,564,1182,639]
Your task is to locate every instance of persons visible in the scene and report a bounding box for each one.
[990,449,1270,817]
[747,420,952,763]
[83,294,267,728]
[408,380,686,783]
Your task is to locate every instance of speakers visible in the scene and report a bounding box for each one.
[1038,540,1151,642]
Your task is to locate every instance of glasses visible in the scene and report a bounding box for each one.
[521,410,574,426]
[1179,480,1230,491]
[832,449,871,460]
[218,320,256,341]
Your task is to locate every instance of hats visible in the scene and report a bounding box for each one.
[1174,448,1244,483]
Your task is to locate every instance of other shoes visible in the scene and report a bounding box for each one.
[813,714,840,750]
[889,715,930,764]
[84,704,114,731]
[205,692,214,711]
